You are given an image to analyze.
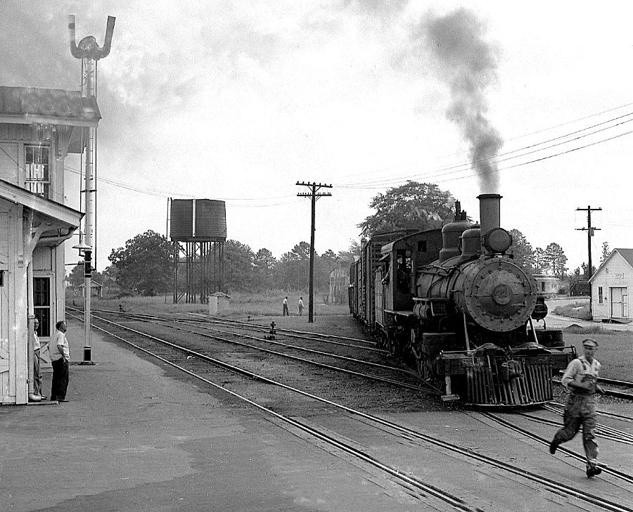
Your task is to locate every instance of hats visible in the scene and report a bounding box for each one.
[582,338,599,347]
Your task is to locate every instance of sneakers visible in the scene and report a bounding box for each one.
[587,467,602,476]
[550,439,558,454]
[61,399,68,402]
[42,394,47,400]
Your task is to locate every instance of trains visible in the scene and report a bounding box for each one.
[346,194,579,411]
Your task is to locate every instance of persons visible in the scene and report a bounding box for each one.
[551,338,602,476]
[282,296,289,316]
[33,318,47,400]
[47,320,71,402]
[298,297,304,316]
